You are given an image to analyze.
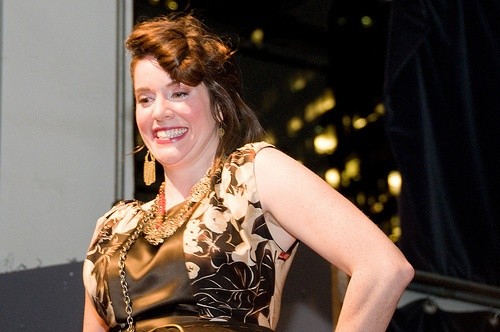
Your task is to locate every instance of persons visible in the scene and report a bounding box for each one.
[81,16,416,332]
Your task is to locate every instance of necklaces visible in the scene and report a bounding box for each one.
[142,161,221,246]
